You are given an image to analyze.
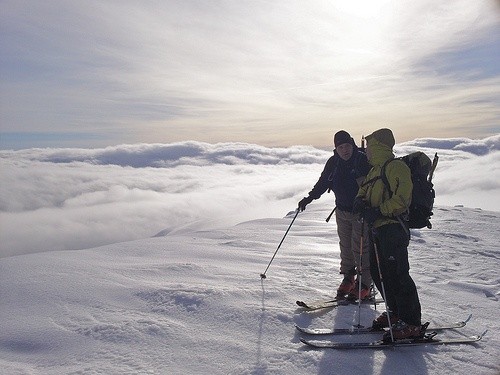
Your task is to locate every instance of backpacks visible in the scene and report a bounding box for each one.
[380,151,435,229]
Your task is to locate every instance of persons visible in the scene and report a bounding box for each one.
[299,131,372,303]
[353,129,421,343]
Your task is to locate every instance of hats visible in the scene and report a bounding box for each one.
[334,130,353,148]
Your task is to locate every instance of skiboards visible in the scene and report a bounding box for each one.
[292,313,488,350]
[296,291,385,309]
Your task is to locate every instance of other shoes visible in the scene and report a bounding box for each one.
[338,276,355,295]
[349,286,370,299]
[377,310,398,325]
[389,320,421,338]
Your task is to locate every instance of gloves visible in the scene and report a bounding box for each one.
[359,205,382,224]
[354,196,370,215]
[298,197,309,212]
